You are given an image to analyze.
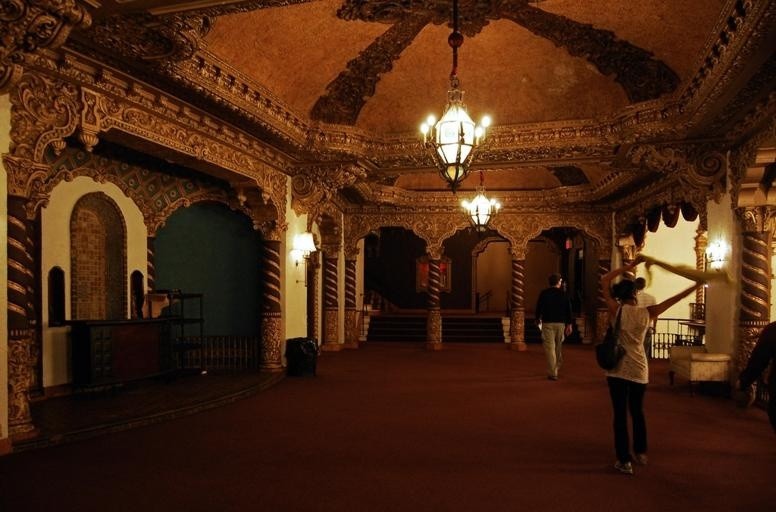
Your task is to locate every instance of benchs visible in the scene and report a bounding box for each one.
[667,344,731,398]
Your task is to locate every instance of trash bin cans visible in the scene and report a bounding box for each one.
[286,337,316,377]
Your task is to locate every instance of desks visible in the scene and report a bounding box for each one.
[678,320,706,347]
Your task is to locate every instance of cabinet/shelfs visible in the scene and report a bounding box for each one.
[149,291,205,389]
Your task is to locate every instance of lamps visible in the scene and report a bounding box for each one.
[420,2,504,235]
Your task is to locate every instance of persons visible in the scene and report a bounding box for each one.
[535,273,573,381]
[634,278,657,357]
[736,322,776,432]
[594,256,704,475]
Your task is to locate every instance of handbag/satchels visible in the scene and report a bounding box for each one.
[593,305,626,370]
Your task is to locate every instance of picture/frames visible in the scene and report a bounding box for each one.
[414,254,454,293]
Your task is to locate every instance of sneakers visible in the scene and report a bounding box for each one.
[632,452,647,465]
[614,460,633,475]
